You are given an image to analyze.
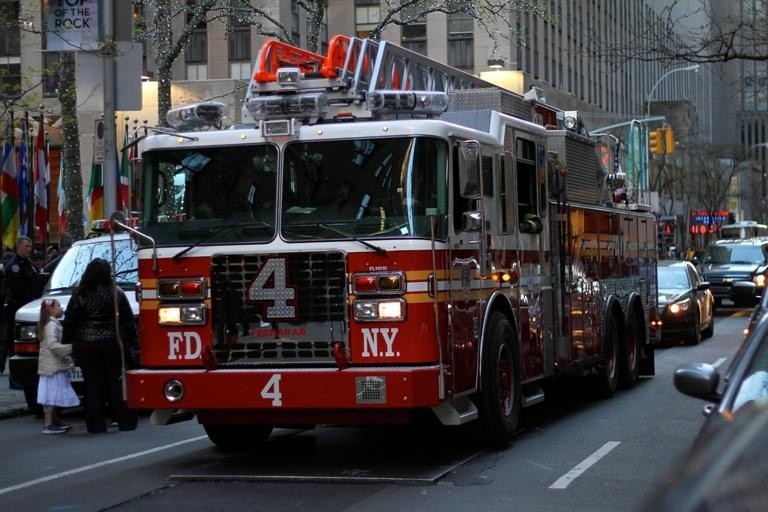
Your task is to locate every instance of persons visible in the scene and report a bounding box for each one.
[37,298,81,434]
[61,257,138,434]
[0,235,42,375]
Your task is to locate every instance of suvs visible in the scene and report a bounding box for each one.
[696,235,768,313]
[8,212,193,426]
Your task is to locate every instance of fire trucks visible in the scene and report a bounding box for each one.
[113,32,661,451]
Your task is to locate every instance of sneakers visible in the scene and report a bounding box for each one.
[42,418,71,434]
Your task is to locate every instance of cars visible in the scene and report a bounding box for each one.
[670,310,768,512]
[657,257,717,350]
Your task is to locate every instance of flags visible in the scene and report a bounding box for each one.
[0,112,130,251]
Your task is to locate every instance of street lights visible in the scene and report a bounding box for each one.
[640,62,705,215]
